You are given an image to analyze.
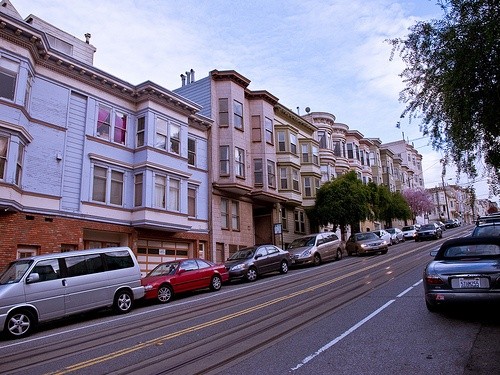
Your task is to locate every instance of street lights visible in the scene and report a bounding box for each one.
[441,175,452,220]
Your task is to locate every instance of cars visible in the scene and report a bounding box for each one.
[345,232,389,256]
[429,220,446,231]
[415,223,443,241]
[471,222,500,239]
[401,225,417,240]
[373,229,392,246]
[444,219,457,229]
[475,216,500,225]
[454,219,460,227]
[224,245,290,282]
[141,258,230,304]
[423,239,500,312]
[489,213,500,216]
[385,228,406,244]
[415,224,421,230]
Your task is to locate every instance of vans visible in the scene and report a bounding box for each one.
[0,247,145,339]
[286,232,344,266]
